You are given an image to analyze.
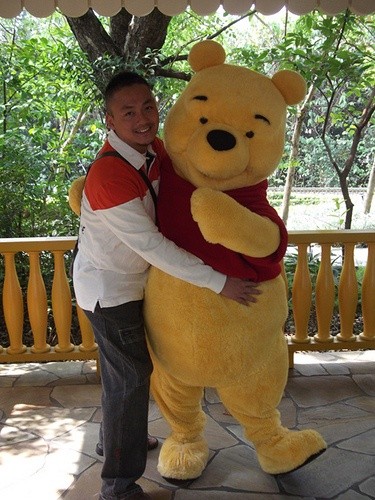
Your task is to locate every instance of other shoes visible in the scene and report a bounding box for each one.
[96,433,158,456]
[99,484,152,500]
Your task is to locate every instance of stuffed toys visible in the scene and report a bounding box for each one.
[70,41,327,485]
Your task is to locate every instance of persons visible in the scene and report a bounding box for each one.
[73,72,263,500]
